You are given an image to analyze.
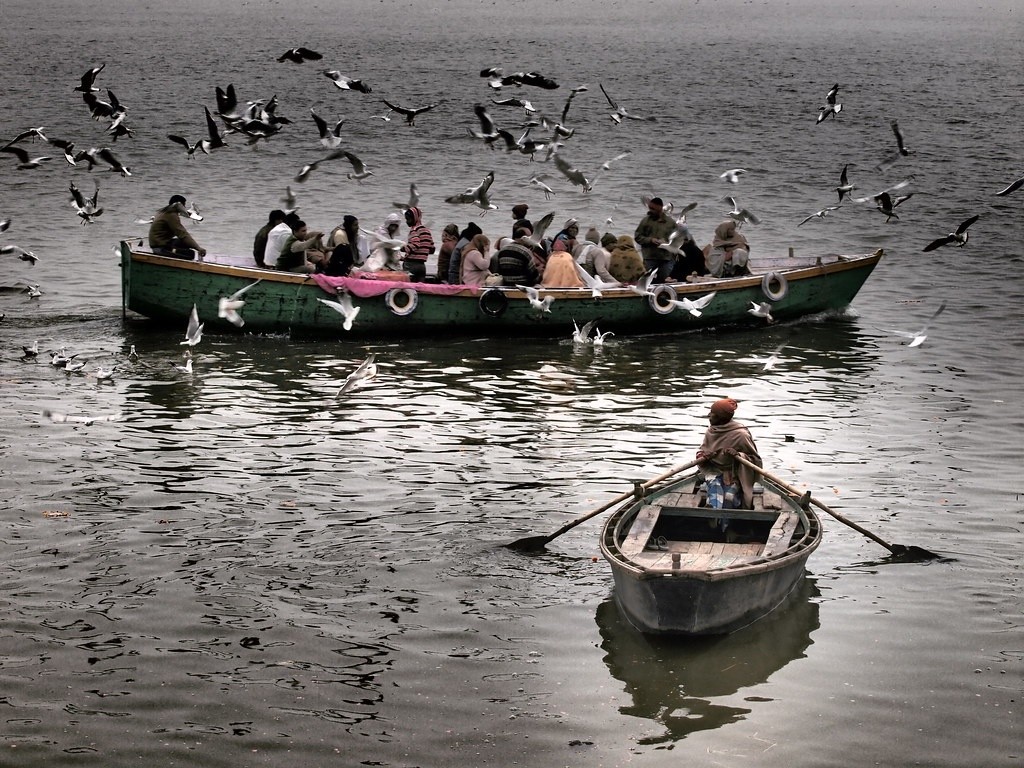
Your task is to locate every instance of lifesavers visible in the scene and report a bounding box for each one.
[385,288,419,316]
[648,283,678,314]
[762,271,788,302]
[479,288,508,318]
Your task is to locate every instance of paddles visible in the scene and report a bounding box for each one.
[502,455,705,551]
[730,452,940,560]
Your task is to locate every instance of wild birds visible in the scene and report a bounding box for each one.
[179,302,204,346]
[665,291,716,317]
[94,366,116,379]
[514,284,553,317]
[0,36,663,266]
[715,81,1024,230]
[337,356,377,397]
[183,351,192,358]
[0,283,89,371]
[128,345,139,364]
[570,259,622,296]
[168,359,193,374]
[592,327,614,344]
[315,280,361,330]
[922,214,980,252]
[874,306,947,347]
[572,317,602,344]
[625,267,659,295]
[748,301,774,320]
[218,279,262,327]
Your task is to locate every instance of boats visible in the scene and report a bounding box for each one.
[117,239,884,337]
[599,460,823,638]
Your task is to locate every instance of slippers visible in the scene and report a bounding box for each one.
[656,536,669,550]
[645,536,658,550]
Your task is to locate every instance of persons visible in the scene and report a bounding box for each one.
[393,206,436,283]
[511,203,534,240]
[610,236,643,284]
[571,227,621,284]
[636,197,677,284]
[449,221,483,284]
[599,232,617,282]
[695,398,763,508]
[254,210,704,284]
[701,221,750,278]
[459,233,500,286]
[148,193,208,259]
[326,215,360,264]
[437,223,461,282]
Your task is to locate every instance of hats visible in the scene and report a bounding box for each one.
[601,233,617,247]
[463,222,482,242]
[711,399,737,420]
[585,228,599,244]
[512,204,529,219]
[343,215,357,225]
